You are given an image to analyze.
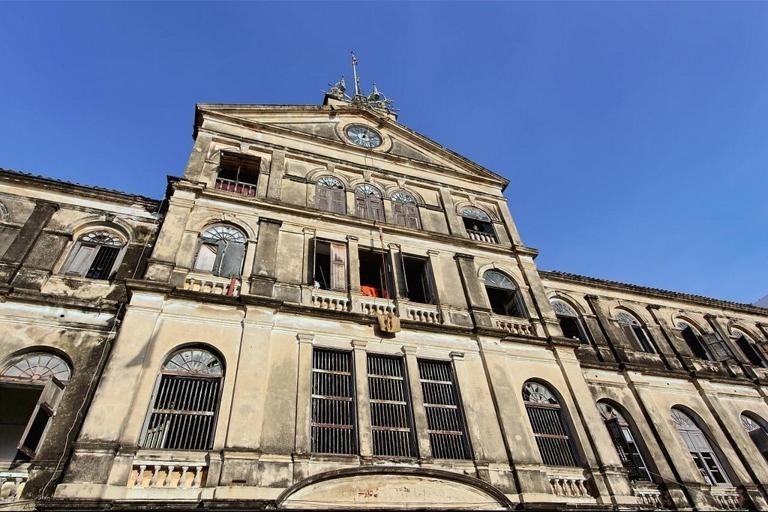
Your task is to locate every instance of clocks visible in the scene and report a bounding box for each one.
[341,123,382,149]
